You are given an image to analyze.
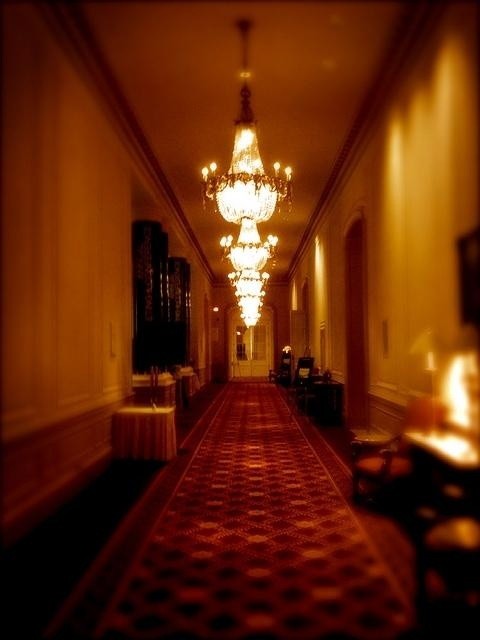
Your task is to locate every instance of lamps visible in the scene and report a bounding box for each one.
[198,17,293,327]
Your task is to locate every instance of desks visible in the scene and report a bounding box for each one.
[112,407,176,461]
[402,431,480,540]
[306,375,343,426]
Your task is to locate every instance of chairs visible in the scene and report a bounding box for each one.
[269,369,288,384]
[349,397,449,512]
